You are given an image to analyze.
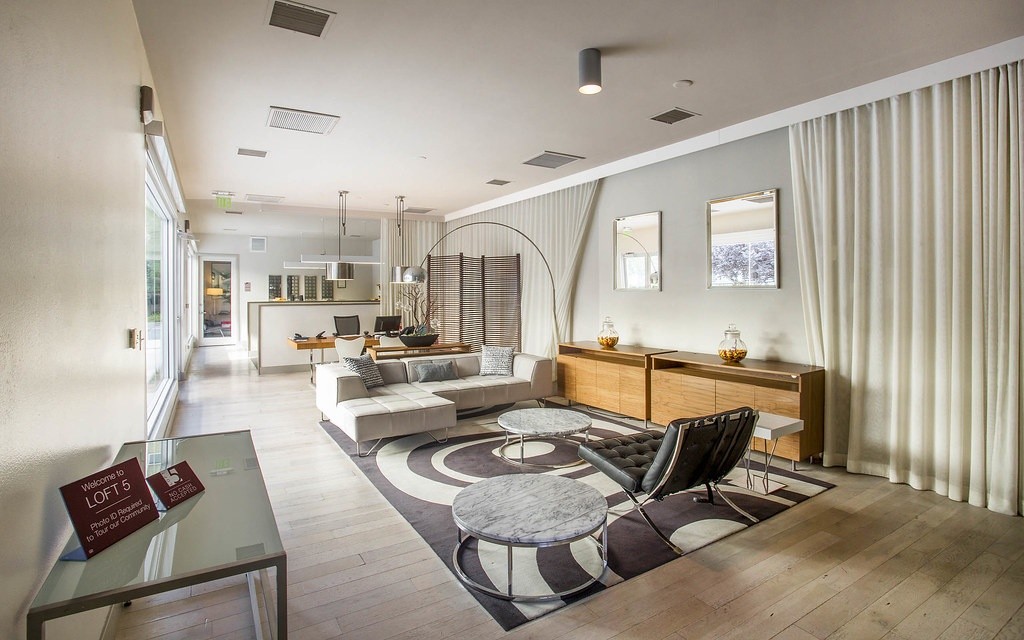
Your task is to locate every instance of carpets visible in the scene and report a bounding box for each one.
[318,396,838,632]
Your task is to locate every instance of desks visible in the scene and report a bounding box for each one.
[23,428,287,640]
[287,334,381,384]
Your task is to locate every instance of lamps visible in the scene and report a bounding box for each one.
[389,196,411,283]
[207,288,223,315]
[578,48,602,95]
[402,222,560,342]
[324,191,354,281]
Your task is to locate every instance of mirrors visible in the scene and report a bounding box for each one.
[612,211,663,292]
[707,188,779,290]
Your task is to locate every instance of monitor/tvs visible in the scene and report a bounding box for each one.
[374,315,401,336]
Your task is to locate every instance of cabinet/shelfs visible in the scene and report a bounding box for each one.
[556,341,827,472]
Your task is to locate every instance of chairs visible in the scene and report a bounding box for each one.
[202,318,225,337]
[334,315,407,358]
[577,406,760,557]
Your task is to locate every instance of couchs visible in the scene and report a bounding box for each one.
[315,351,554,457]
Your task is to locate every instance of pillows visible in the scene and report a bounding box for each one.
[478,344,515,377]
[341,354,385,388]
[413,360,460,383]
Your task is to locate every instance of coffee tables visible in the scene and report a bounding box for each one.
[365,342,472,360]
[727,410,805,497]
[451,472,610,603]
[497,408,593,469]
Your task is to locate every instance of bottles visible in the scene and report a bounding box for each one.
[597,316,618,348]
[718,324,747,363]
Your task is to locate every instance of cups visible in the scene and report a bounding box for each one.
[290,295,294,301]
[299,294,303,301]
